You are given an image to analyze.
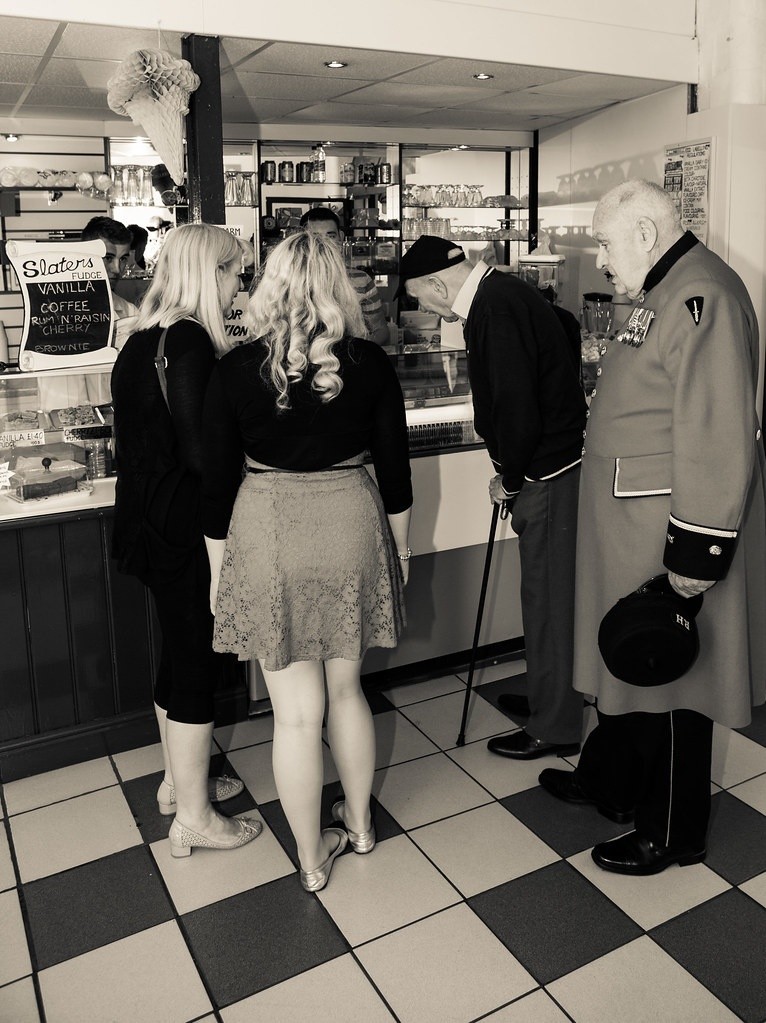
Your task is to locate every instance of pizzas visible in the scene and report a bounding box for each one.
[58,404,96,426]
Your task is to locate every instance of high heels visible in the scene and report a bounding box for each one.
[157,775,244,816]
[168,816,263,858]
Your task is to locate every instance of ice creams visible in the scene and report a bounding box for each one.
[107,48,199,187]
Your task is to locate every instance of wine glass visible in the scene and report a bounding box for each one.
[107,165,156,206]
[497,219,525,240]
[402,184,484,207]
[452,226,498,240]
[224,170,256,207]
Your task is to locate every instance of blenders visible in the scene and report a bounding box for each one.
[579,292,616,341]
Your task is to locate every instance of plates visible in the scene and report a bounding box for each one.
[0,169,111,191]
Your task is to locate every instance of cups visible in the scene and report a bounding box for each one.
[403,218,451,240]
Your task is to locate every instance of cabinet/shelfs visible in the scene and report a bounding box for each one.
[260,140,540,341]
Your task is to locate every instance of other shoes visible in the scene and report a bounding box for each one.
[333,798,376,854]
[299,820,349,892]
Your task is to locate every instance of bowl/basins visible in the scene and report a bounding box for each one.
[400,308,441,343]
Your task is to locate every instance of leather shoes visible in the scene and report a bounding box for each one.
[537,766,636,826]
[591,830,707,876]
[488,729,582,759]
[496,691,532,717]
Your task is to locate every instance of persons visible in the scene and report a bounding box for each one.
[124,224,148,273]
[220,229,412,894]
[109,222,266,858]
[395,234,588,761]
[540,179,766,874]
[36,217,139,410]
[300,207,392,347]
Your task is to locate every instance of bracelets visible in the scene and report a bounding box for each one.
[398,548,411,560]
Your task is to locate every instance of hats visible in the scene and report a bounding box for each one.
[392,234,466,303]
[597,573,705,688]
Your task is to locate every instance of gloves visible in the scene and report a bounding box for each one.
[669,570,717,599]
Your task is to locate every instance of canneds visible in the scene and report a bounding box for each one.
[263,160,392,185]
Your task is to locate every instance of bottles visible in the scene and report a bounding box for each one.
[315,144,326,183]
[309,145,314,182]
[386,316,399,345]
[87,439,106,477]
